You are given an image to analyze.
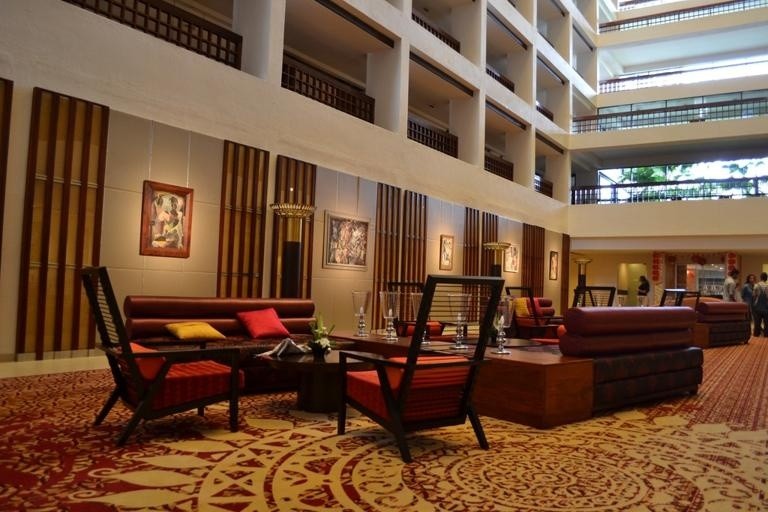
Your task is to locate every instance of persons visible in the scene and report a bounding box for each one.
[721,268,740,303]
[751,271,767,339]
[741,274,763,334]
[635,275,650,308]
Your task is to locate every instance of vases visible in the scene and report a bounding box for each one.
[310,344,326,361]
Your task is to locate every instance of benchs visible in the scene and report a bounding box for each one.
[688,302,752,349]
[559,307,703,417]
[122,295,353,394]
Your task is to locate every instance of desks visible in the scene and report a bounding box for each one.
[324,331,594,430]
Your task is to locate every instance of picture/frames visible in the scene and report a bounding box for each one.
[549,251,559,283]
[322,208,373,271]
[139,180,193,258]
[439,234,454,271]
[502,242,520,274]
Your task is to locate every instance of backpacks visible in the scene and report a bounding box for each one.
[752,294,767,315]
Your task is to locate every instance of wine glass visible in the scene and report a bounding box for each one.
[410,293,431,344]
[379,292,402,340]
[351,290,372,337]
[448,293,473,350]
[487,296,516,355]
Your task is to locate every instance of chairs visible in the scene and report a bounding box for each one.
[386,281,444,336]
[338,275,505,463]
[529,287,616,344]
[658,287,687,308]
[506,286,550,327]
[81,267,246,446]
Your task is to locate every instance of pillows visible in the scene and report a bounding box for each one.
[236,306,292,340]
[527,301,544,317]
[509,297,530,317]
[167,321,226,344]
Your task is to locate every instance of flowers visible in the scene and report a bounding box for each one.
[309,310,336,351]
